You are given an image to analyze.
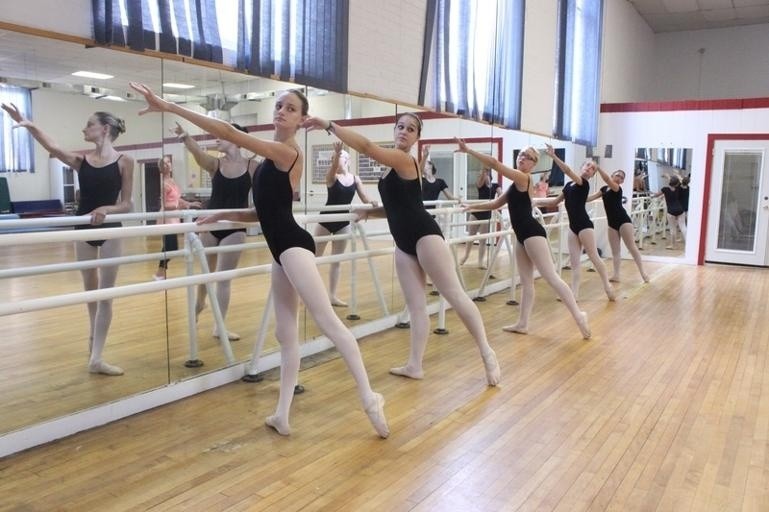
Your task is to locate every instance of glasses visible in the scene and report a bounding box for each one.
[520,151,536,162]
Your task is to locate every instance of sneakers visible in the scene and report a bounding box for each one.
[266,417,290,435]
[611,277,620,283]
[642,272,650,282]
[578,312,591,338]
[214,330,240,340]
[89,362,123,376]
[391,367,421,380]
[484,351,500,386]
[331,298,347,307]
[607,285,616,300]
[367,394,389,438]
[502,325,529,334]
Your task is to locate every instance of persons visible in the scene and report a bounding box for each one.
[583,163,653,286]
[1,100,134,377]
[458,160,502,271]
[533,172,551,212]
[720,191,749,247]
[304,110,501,388]
[128,80,391,440]
[154,155,201,282]
[416,144,462,287]
[168,118,261,342]
[452,135,593,341]
[632,162,690,253]
[532,144,615,304]
[310,140,379,310]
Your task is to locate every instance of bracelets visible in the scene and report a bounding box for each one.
[484,168,490,172]
[325,121,335,137]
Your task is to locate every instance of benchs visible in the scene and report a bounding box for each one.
[11,200,66,216]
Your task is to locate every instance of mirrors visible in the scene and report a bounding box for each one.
[632,145,695,256]
[0,21,590,447]
